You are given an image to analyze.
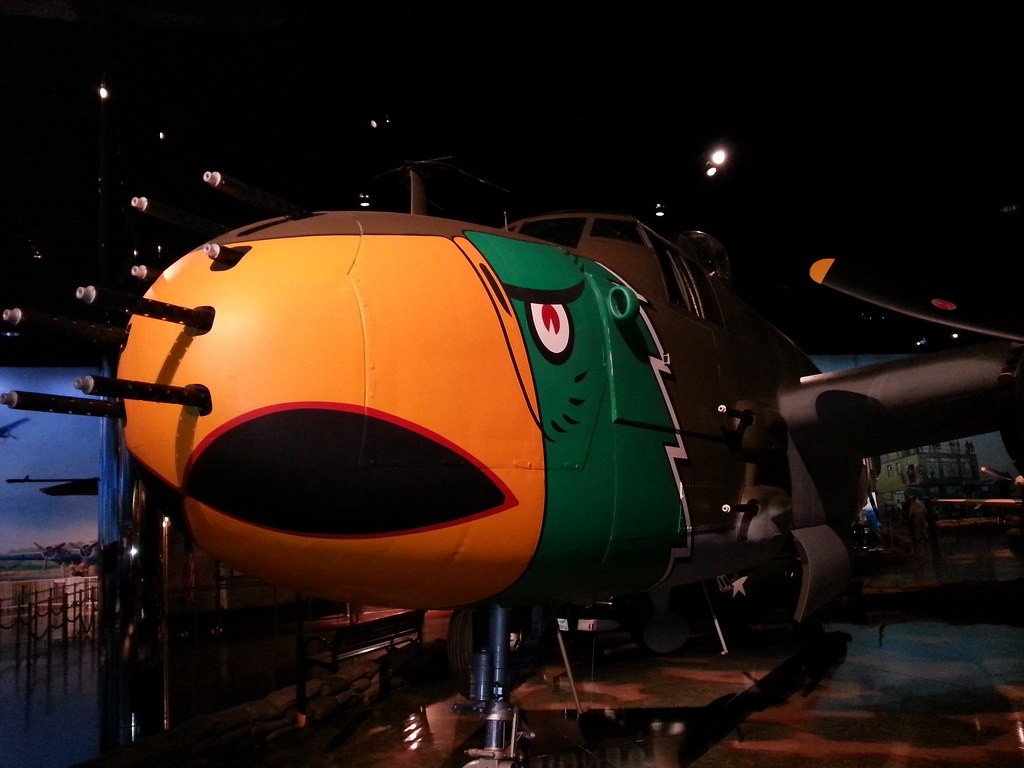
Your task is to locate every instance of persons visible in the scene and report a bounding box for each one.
[910,497,930,541]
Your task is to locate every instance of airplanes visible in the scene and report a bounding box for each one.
[0,149,1024,767]
